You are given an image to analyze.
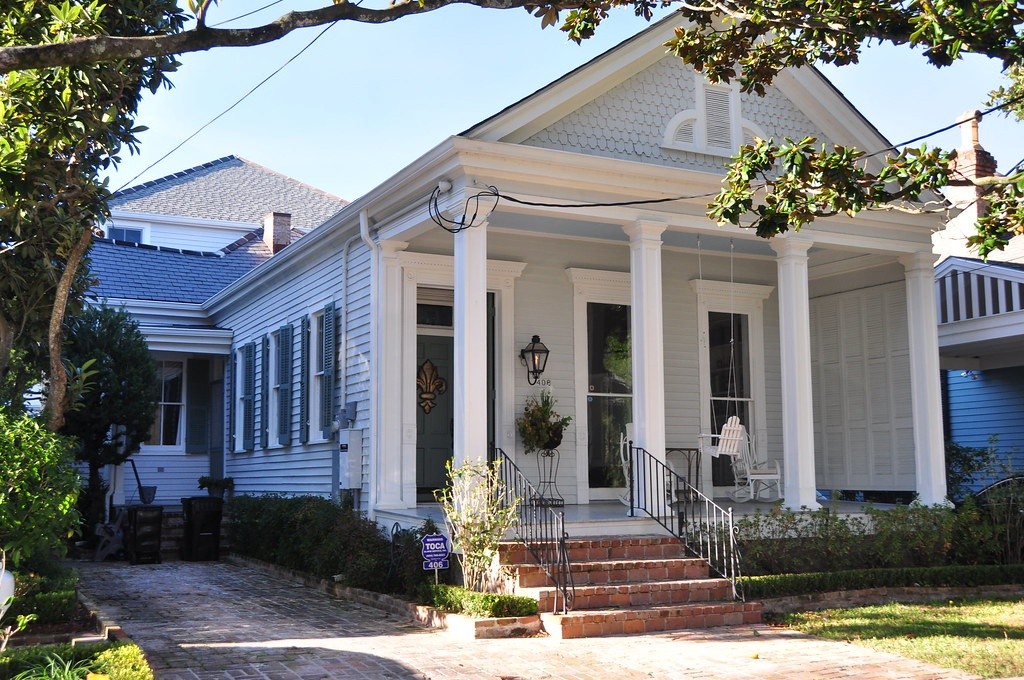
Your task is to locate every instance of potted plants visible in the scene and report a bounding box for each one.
[515,388,572,455]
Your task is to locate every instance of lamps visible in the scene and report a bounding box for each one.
[519,335,550,385]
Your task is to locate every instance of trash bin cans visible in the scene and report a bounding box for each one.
[180,496,224,560]
[125,504,164,565]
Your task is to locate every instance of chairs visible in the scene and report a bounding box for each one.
[726,424,783,503]
[616,432,678,507]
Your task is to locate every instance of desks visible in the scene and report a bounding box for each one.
[665,448,699,501]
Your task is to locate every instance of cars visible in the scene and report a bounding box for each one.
[953,476,1024,513]
